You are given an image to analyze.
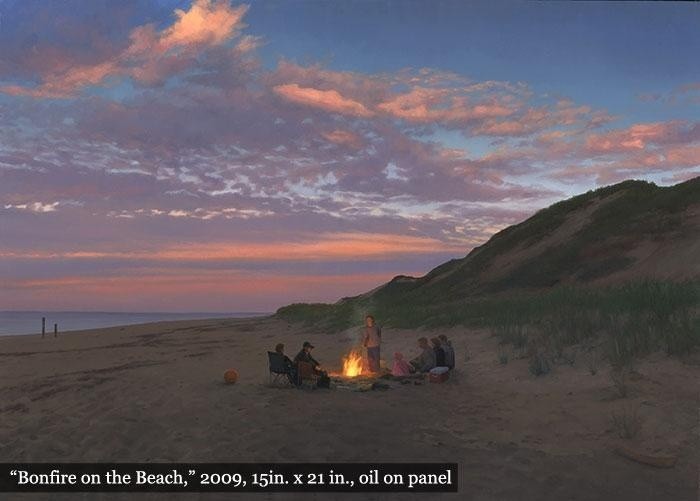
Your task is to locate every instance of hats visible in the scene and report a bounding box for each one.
[302,341,315,348]
[393,352,403,359]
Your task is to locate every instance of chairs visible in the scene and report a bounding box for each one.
[296,360,326,391]
[267,351,294,390]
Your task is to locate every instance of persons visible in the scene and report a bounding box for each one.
[362,314,382,373]
[392,334,455,377]
[276,340,323,384]
